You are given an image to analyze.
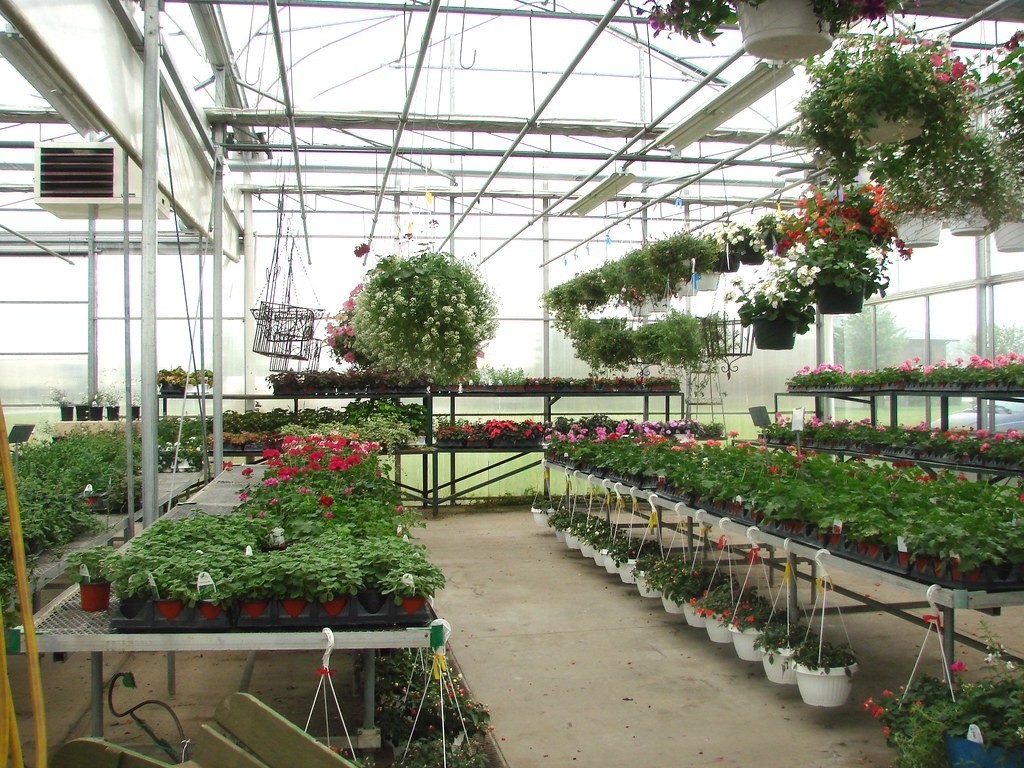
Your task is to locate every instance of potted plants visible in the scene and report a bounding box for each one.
[64,523,445,621]
[449,366,524,392]
[573,324,738,370]
[153,363,214,396]
[82,475,142,514]
[48,380,140,422]
[537,254,607,311]
[266,365,430,395]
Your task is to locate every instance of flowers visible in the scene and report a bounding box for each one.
[699,173,914,320]
[158,436,203,454]
[560,514,764,627]
[237,428,386,520]
[855,639,1016,738]
[630,0,1024,110]
[322,647,509,768]
[790,351,1024,379]
[524,373,677,384]
[331,248,500,362]
[435,410,1024,506]
[616,284,647,304]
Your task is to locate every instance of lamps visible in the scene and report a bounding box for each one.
[557,172,637,217]
[660,61,796,152]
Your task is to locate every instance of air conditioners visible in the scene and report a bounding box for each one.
[33,140,170,222]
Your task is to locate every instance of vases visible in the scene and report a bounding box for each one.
[614,555,639,585]
[738,245,766,266]
[791,658,859,707]
[531,505,565,528]
[813,277,866,313]
[734,0,834,60]
[492,435,516,448]
[761,645,803,685]
[894,210,943,248]
[159,457,198,472]
[680,592,707,630]
[526,384,674,390]
[749,314,798,350]
[466,435,491,448]
[660,585,685,615]
[594,547,616,567]
[948,202,990,236]
[672,259,693,271]
[994,220,1024,253]
[779,517,983,584]
[514,436,541,449]
[563,528,585,550]
[629,291,673,317]
[694,271,721,292]
[554,526,569,544]
[579,538,601,558]
[633,569,663,599]
[601,550,620,574]
[705,613,734,644]
[860,99,926,145]
[436,436,466,449]
[728,623,765,661]
[674,277,698,297]
[943,728,1024,768]
